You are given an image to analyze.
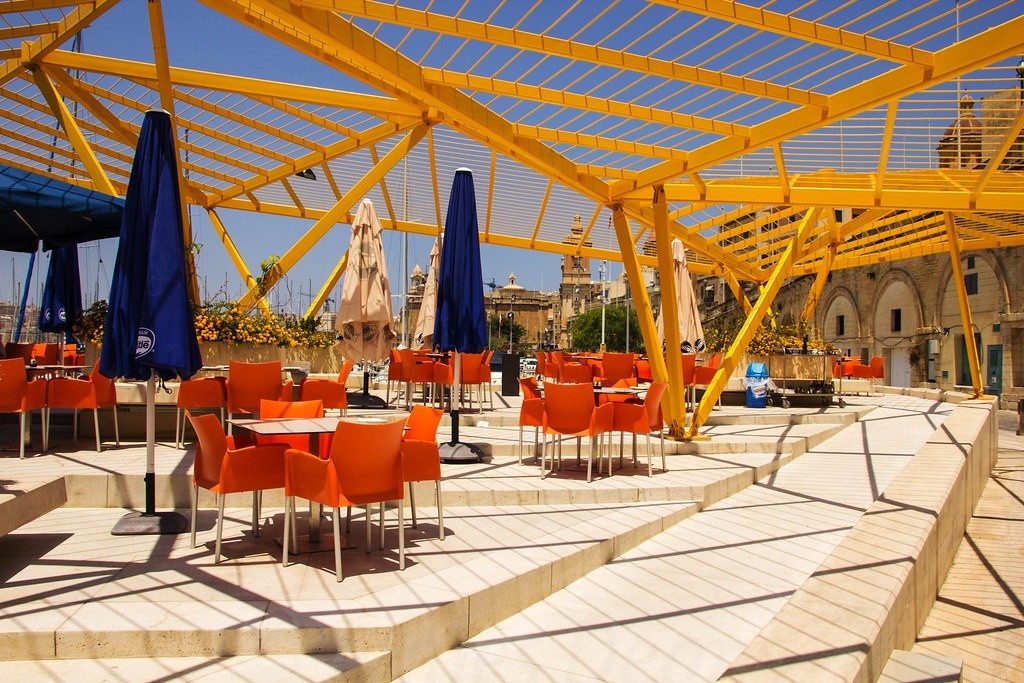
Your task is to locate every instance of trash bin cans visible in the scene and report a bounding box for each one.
[745,362,769,408]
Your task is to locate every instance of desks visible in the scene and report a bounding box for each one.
[566,387,645,474]
[226,418,388,555]
[0,365,91,452]
[426,354,466,412]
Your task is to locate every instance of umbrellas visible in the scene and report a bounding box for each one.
[333,198,397,394]
[656,238,706,355]
[98,108,203,516]
[415,167,489,442]
[38,209,82,366]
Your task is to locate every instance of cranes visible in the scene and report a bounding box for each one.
[298,292,334,313]
[483,277,504,293]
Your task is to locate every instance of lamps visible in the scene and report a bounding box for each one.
[296,168,317,181]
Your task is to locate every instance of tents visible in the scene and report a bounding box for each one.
[0,163,126,343]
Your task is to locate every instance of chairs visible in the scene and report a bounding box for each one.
[833,355,885,398]
[387,348,494,414]
[175,359,446,580]
[517,350,722,483]
[0,342,120,458]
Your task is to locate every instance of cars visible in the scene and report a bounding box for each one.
[520,358,538,371]
